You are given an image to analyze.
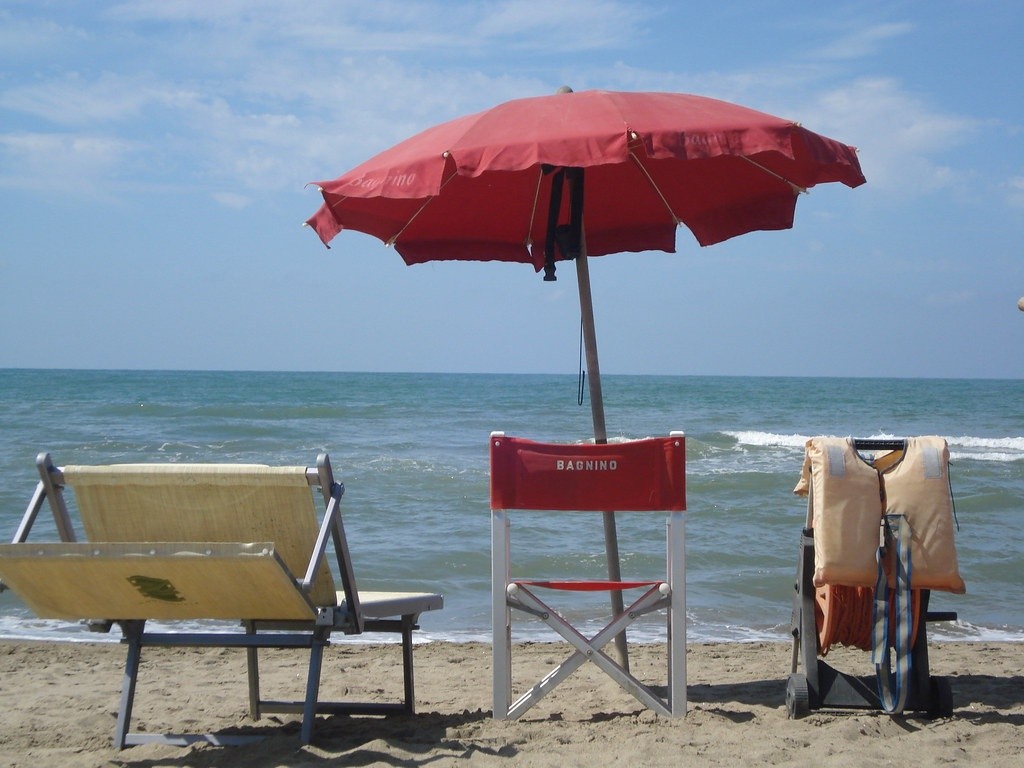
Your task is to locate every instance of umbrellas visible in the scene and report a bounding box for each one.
[301,86,866,673]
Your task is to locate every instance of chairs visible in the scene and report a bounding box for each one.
[489,430,687,722]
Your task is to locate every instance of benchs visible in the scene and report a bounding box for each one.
[35,452,445,749]
